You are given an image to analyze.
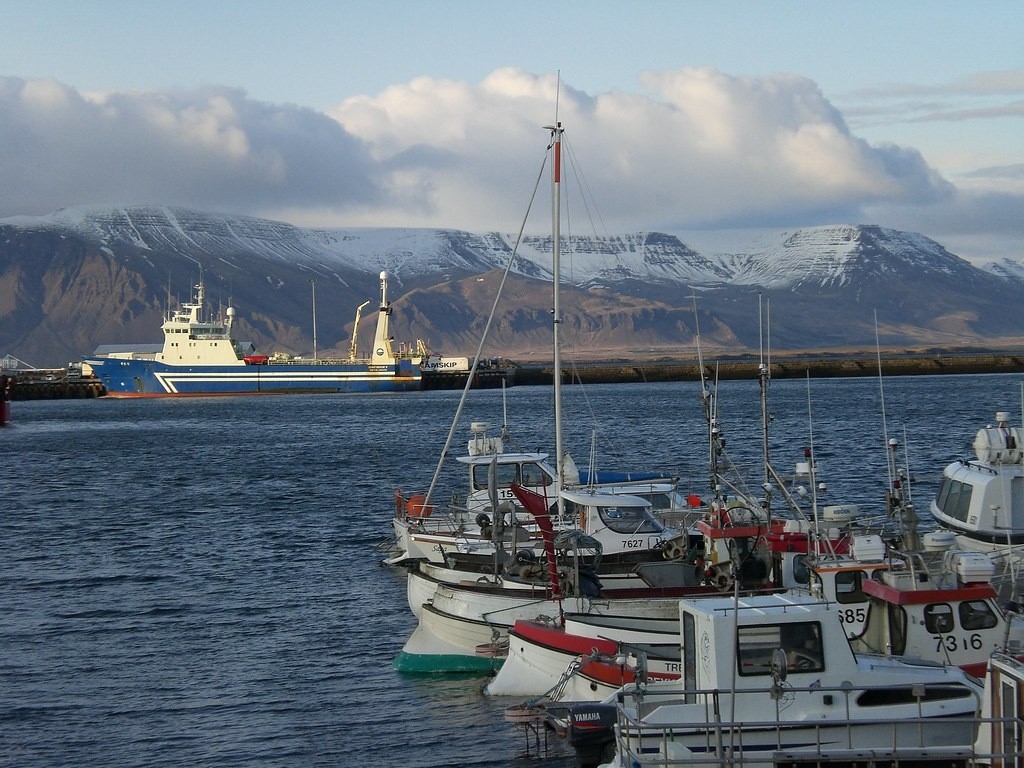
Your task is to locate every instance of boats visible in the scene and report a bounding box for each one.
[0,255,532,395]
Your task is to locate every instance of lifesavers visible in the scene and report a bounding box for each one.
[396,489,402,515]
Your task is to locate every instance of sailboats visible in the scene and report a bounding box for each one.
[370,60,1020,768]
